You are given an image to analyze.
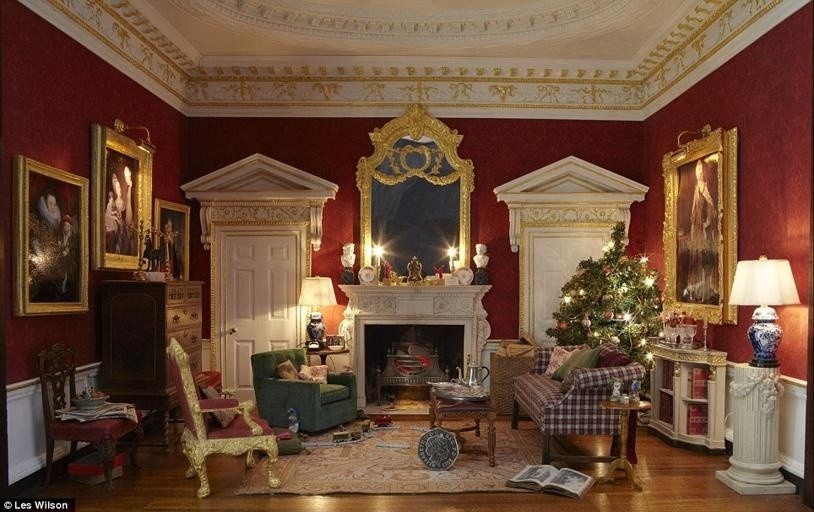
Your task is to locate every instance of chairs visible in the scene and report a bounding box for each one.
[165,338,281,499]
[30,337,142,492]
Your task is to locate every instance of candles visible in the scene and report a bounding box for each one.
[702,306,710,328]
[375,256,380,265]
[450,257,454,268]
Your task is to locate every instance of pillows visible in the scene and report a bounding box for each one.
[552,347,602,382]
[299,365,328,384]
[545,346,579,376]
[276,360,299,379]
[597,342,632,368]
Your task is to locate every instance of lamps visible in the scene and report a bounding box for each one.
[728,255,802,368]
[298,277,337,341]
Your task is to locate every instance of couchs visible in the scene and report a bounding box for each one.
[250,348,357,433]
[512,343,646,465]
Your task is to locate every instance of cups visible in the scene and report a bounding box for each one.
[383,278,392,287]
[426,276,437,281]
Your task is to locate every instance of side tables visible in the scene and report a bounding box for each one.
[306,349,349,366]
[599,401,652,491]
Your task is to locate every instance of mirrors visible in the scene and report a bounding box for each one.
[356,103,474,285]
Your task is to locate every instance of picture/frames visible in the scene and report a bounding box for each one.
[11,154,88,319]
[90,118,156,274]
[661,124,739,328]
[153,198,189,281]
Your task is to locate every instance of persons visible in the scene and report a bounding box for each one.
[106,190,121,233]
[123,167,137,255]
[683,160,719,302]
[112,172,127,254]
[40,194,61,228]
[57,214,81,302]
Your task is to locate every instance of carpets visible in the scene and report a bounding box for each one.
[234,420,573,494]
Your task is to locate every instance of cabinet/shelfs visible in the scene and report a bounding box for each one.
[97,279,210,412]
[648,343,728,456]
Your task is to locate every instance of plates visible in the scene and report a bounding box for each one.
[359,267,378,284]
[432,387,492,402]
[455,268,475,286]
[433,382,454,387]
[329,346,344,351]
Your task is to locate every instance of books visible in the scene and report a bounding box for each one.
[505,464,597,501]
[691,366,707,399]
[662,359,673,391]
[687,403,708,435]
[659,391,673,427]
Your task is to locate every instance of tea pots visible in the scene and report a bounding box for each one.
[457,360,491,388]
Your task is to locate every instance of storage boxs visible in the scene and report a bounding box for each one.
[490,341,534,415]
